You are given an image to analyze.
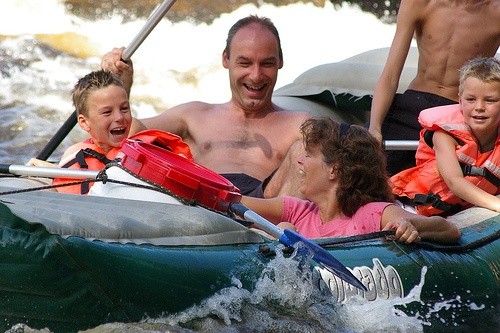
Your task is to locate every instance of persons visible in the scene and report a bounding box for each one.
[235,115,461,246]
[25,68,195,193]
[101,14,313,228]
[369,0,500,179]
[389,54,500,217]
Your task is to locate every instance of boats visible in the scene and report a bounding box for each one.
[0,41,500,333]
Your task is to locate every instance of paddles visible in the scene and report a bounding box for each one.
[36,0,178,163]
[382,140,420,152]
[0,162,96,183]
[232,202,369,293]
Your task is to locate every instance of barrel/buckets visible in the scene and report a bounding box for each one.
[87,139,242,212]
